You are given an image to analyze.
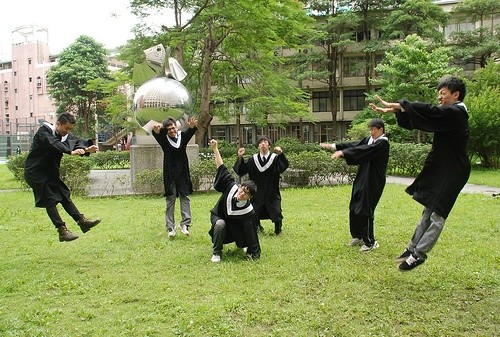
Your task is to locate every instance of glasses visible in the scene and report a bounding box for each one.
[240,186,252,198]
[167,127,176,131]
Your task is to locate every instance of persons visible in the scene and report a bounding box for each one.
[24,111,101,242]
[152,117,198,237]
[369,75,472,271]
[232,135,289,235]
[319,118,390,253]
[17,145,21,154]
[207,139,261,263]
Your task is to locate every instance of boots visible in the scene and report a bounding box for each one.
[77,215,102,233]
[57,225,79,242]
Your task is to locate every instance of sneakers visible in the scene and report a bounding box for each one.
[396,248,413,260]
[397,254,425,271]
[167,229,176,238]
[210,254,221,262]
[360,240,380,252]
[350,238,364,246]
[178,222,189,235]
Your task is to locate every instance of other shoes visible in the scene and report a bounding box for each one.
[257,225,264,233]
[270,231,277,236]
